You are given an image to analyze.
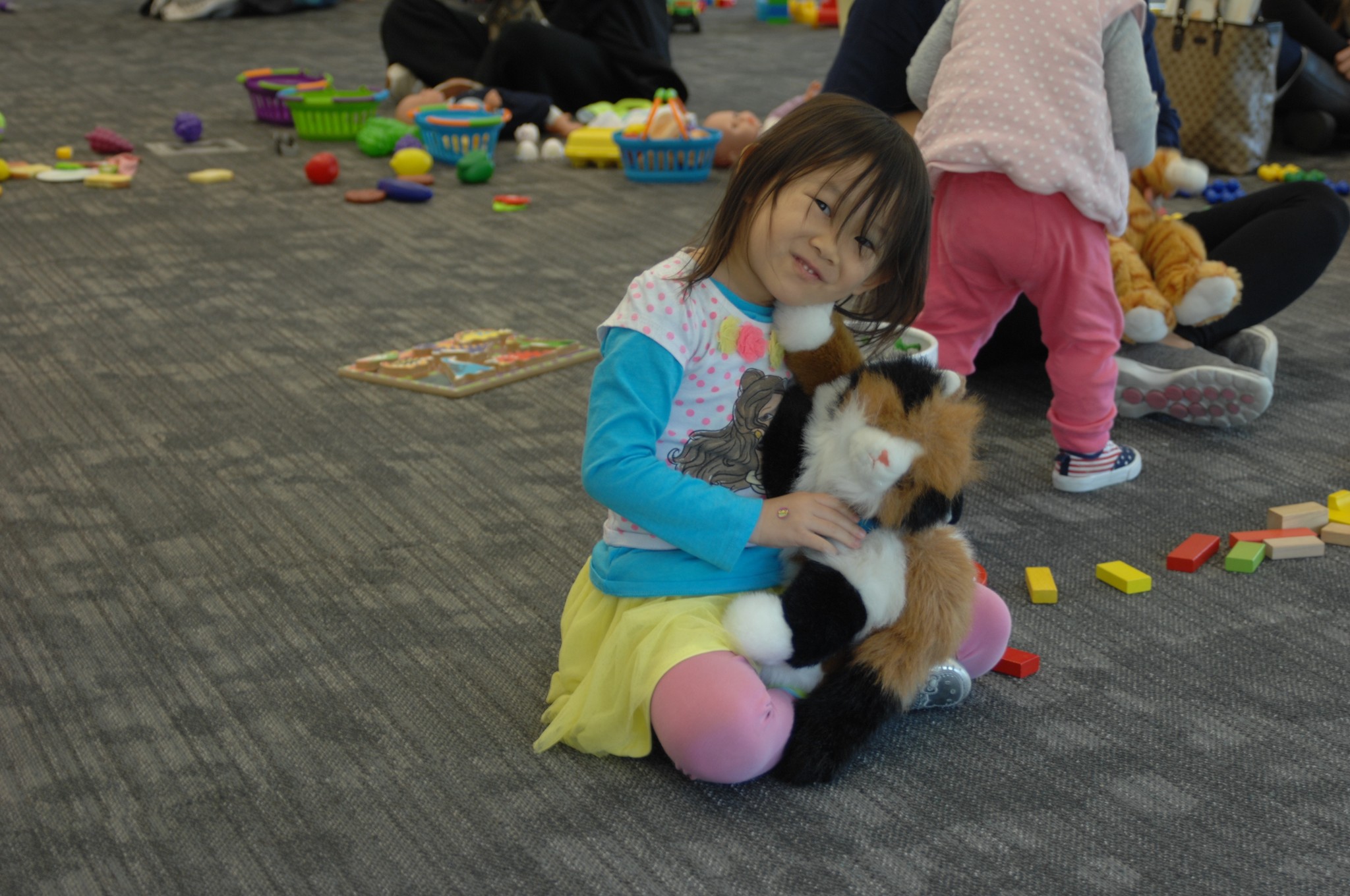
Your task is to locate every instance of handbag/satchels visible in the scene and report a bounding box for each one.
[1148,0,1283,175]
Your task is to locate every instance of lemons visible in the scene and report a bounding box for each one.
[1256,164,1326,183]
[389,146,433,175]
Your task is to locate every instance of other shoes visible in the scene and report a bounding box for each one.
[1111,335,1275,426]
[908,660,971,710]
[1212,320,1277,388]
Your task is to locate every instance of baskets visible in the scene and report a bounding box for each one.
[278,80,391,143]
[409,98,514,172]
[613,84,722,186]
[234,62,335,125]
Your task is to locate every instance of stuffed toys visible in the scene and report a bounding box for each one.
[726,297,981,789]
[1104,143,1245,343]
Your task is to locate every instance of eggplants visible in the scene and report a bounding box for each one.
[173,112,202,142]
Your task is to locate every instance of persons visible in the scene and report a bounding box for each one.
[529,97,1016,789]
[381,0,688,145]
[821,0,1350,496]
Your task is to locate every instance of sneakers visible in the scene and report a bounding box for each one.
[1052,440,1142,492]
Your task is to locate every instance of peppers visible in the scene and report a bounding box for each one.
[306,152,338,183]
[84,127,134,152]
[456,150,494,183]
